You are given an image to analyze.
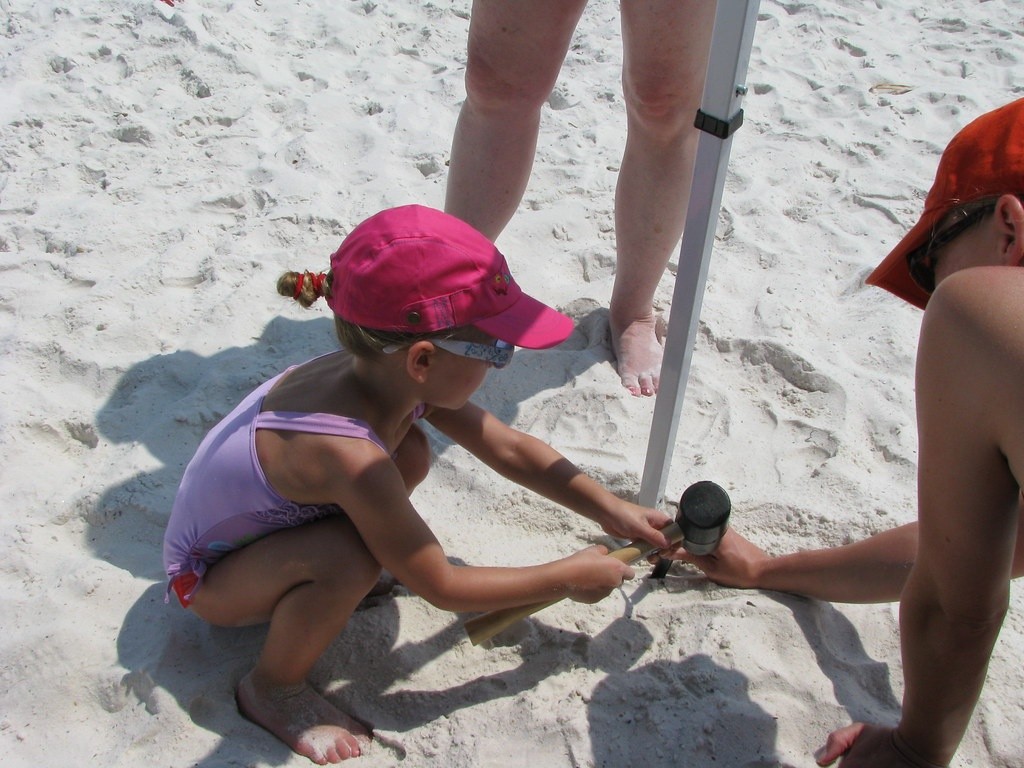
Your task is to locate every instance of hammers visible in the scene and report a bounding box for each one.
[463,480,732,648]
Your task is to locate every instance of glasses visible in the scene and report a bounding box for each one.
[906,202,996,299]
[381,338,515,370]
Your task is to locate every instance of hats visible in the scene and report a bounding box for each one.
[321,204,576,353]
[866,95,1024,314]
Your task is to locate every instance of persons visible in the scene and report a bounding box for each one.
[162,204,682,763]
[445,0,716,395]
[648,98,1024,768]
